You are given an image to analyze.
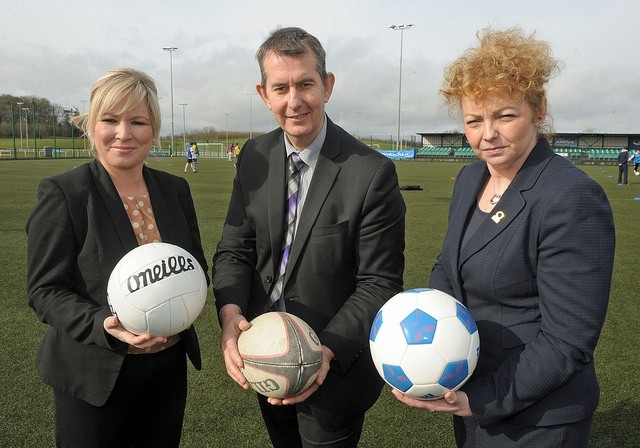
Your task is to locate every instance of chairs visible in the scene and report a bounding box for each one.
[553,146,636,161]
[416,146,479,160]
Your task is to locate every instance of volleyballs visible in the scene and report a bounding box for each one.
[107,241,207,337]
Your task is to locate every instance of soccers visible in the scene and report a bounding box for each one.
[369,286,480,400]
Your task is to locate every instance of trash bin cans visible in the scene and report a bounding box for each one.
[44,146,52,156]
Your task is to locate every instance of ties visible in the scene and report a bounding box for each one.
[278,153,304,275]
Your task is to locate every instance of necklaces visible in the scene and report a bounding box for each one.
[490,176,502,204]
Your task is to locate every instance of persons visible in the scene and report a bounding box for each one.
[617,146,629,185]
[392,30,617,446]
[627,148,640,173]
[24,67,211,446]
[184,141,200,173]
[226,141,240,167]
[211,27,408,446]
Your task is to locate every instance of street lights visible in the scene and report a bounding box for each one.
[224,113,232,154]
[244,93,257,139]
[79,97,88,150]
[16,103,24,149]
[389,24,414,150]
[178,104,187,151]
[22,108,32,149]
[161,48,179,157]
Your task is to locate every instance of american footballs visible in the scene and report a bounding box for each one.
[237,311,323,398]
